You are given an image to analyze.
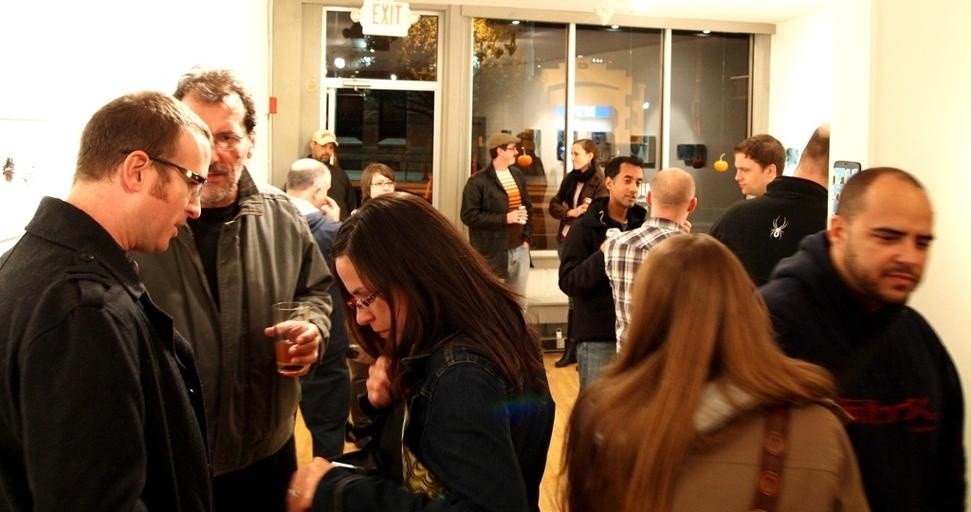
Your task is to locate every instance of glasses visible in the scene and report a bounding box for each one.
[502,145,517,152]
[371,181,396,188]
[116,151,209,198]
[214,128,250,149]
[342,278,400,312]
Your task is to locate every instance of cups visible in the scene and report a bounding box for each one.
[273,302,309,375]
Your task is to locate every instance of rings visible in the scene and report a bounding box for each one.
[287,488,301,496]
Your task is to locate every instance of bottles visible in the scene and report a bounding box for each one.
[519,206,527,226]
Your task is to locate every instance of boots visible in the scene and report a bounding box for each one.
[554,339,579,368]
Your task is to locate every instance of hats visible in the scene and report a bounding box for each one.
[310,128,339,147]
[485,132,522,151]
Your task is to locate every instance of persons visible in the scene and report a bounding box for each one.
[2,91,217,511]
[127,73,335,512]
[548,138,697,385]
[565,231,869,512]
[751,167,967,511]
[511,130,548,251]
[286,196,556,510]
[282,130,395,462]
[710,127,832,287]
[460,132,534,317]
